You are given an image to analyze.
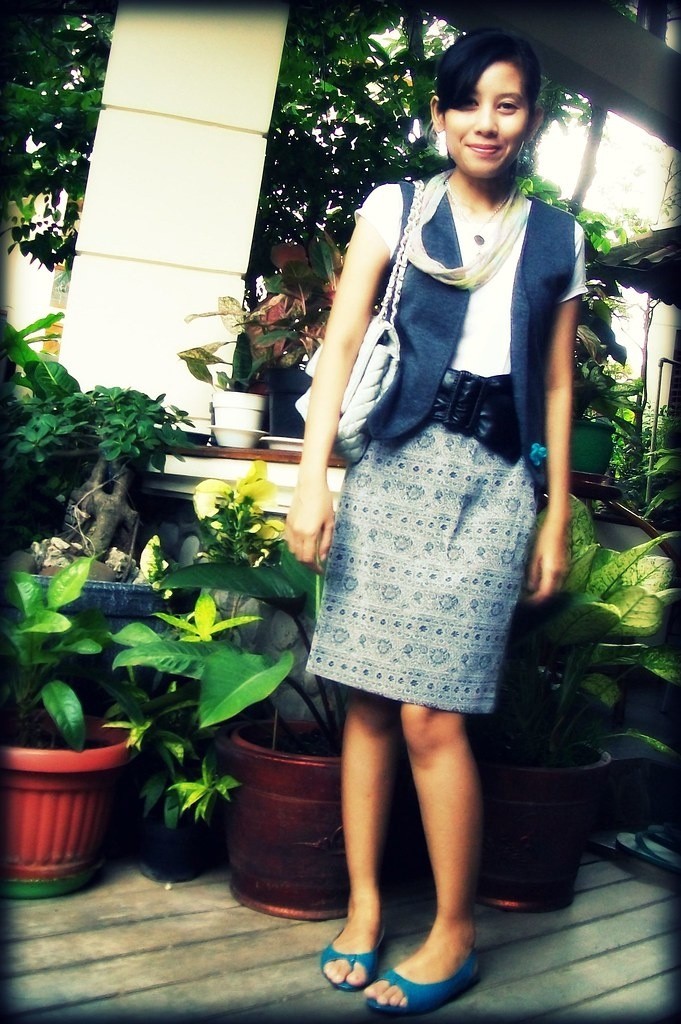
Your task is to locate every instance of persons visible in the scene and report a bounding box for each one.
[283,29,587,1018]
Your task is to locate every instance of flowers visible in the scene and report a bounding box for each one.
[140,459,286,640]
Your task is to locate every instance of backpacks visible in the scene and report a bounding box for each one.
[296,181,423,466]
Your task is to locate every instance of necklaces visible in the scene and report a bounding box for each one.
[445,179,510,247]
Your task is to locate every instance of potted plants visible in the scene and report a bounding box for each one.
[0,300,680,921]
[177,231,346,430]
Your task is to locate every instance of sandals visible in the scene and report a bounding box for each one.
[366,943,481,1014]
[322,920,386,993]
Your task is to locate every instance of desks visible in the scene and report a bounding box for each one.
[164,445,622,519]
